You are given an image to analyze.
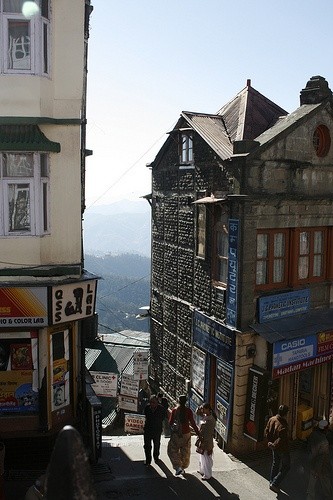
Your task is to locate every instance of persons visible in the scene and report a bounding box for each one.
[304,420,333,500]
[196,403,217,480]
[168,396,200,475]
[264,404,290,492]
[138,382,170,466]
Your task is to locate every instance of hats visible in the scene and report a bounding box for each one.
[319,420,329,429]
[176,395,188,402]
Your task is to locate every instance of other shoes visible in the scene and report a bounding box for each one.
[202,474,212,480]
[145,457,151,465]
[197,470,204,475]
[153,455,159,460]
[269,484,280,493]
[175,468,185,476]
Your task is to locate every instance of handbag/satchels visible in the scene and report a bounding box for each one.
[196,447,204,455]
